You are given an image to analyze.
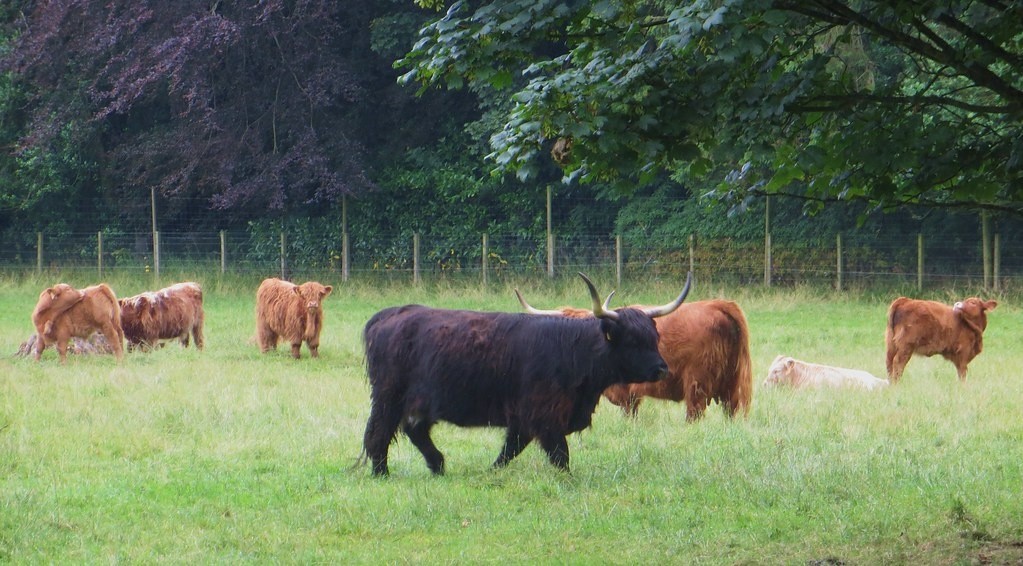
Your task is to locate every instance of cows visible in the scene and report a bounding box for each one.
[886,294,999,380]
[760,353,891,392]
[18,282,205,361]
[363,270,752,478]
[253,277,334,359]
